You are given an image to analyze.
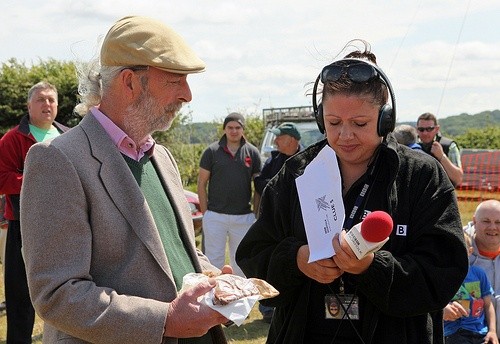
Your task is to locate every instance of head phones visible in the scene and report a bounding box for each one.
[313,58,396,137]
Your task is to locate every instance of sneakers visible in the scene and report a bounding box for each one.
[263,312,273,323]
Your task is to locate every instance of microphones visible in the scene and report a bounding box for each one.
[344,211,395,260]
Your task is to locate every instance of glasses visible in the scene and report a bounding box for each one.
[321,63,388,88]
[417,127,436,132]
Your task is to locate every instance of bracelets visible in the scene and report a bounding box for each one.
[163,324,166,336]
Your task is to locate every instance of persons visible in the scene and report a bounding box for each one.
[198,112,261,327]
[19,16,233,344]
[235,39,469,344]
[0,82,72,344]
[326,295,344,317]
[255,123,304,197]
[442,199,500,344]
[393,124,423,152]
[443,232,499,344]
[416,113,463,189]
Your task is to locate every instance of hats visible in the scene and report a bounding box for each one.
[222,113,244,129]
[100,16,207,74]
[394,125,416,144]
[270,122,301,140]
